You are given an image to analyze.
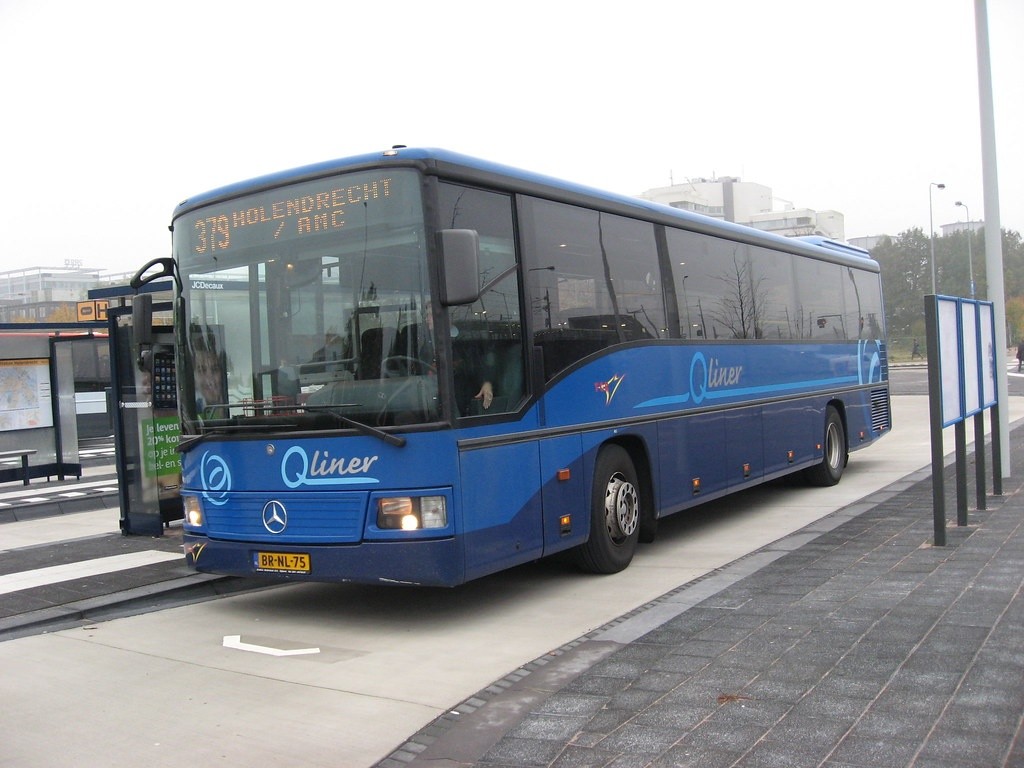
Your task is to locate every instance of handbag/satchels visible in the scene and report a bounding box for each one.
[1016,353,1021,358]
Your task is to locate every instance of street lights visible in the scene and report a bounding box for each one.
[954,201,976,299]
[929,182,945,294]
[683,276,692,340]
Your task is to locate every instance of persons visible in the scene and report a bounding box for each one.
[912,339,923,360]
[1016,337,1024,372]
[195,334,225,420]
[417,300,494,410]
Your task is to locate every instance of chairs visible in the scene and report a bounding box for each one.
[358,323,421,380]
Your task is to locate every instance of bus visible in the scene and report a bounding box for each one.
[130,144,892,590]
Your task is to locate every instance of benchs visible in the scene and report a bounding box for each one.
[0,449,38,486]
